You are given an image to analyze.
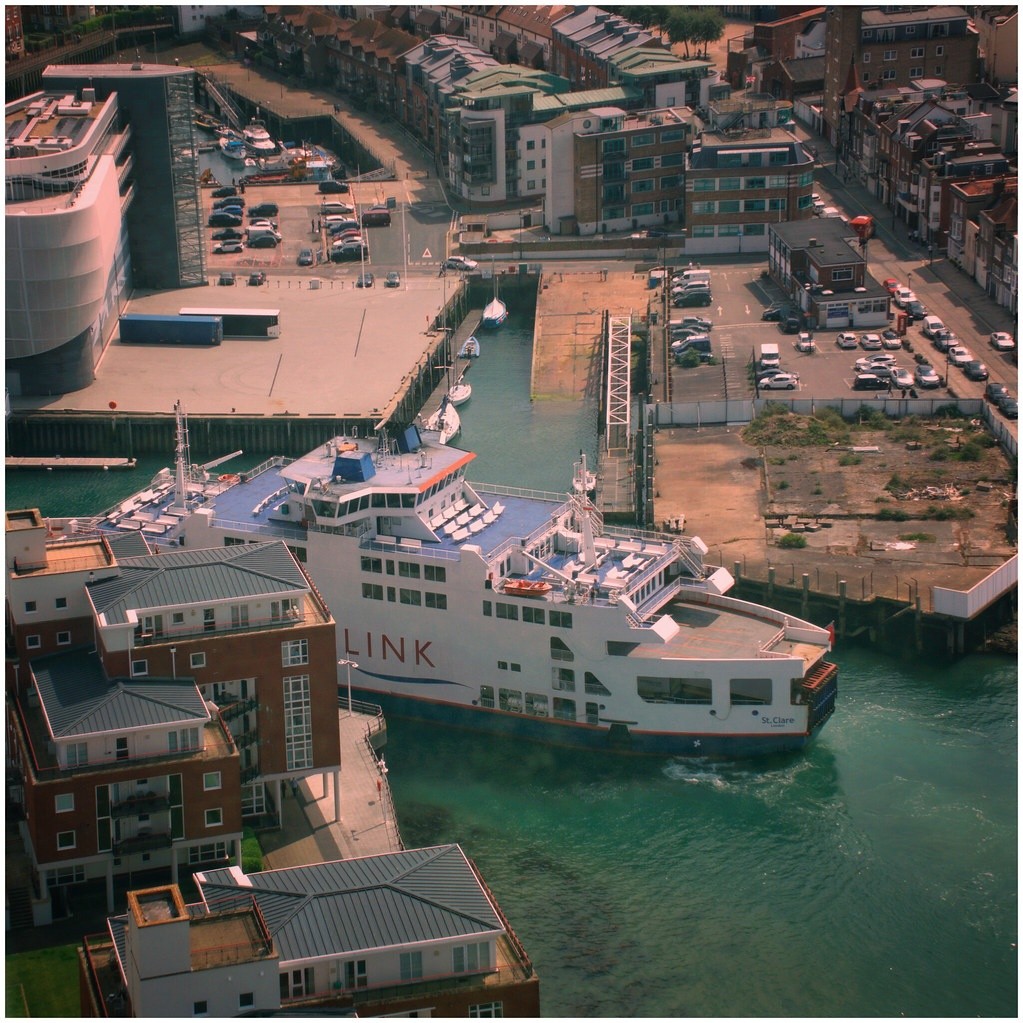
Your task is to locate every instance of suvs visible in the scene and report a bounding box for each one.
[318,180,350,194]
[320,200,354,215]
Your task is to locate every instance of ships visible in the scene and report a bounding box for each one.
[93,401,840,757]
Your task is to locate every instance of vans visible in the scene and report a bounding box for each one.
[357,208,392,228]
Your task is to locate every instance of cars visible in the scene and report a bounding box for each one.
[998,398,1018,421]
[357,272,374,288]
[207,195,283,253]
[323,215,369,262]
[384,271,400,288]
[445,256,477,272]
[990,331,1015,350]
[665,213,990,392]
[296,248,313,266]
[218,271,235,286]
[211,185,236,197]
[249,271,266,285]
[987,381,1010,404]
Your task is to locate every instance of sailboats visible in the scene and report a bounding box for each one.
[571,454,598,495]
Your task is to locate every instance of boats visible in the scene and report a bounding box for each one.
[446,384,472,406]
[504,577,553,598]
[423,394,463,444]
[482,296,508,329]
[459,335,482,360]
[193,107,350,180]
